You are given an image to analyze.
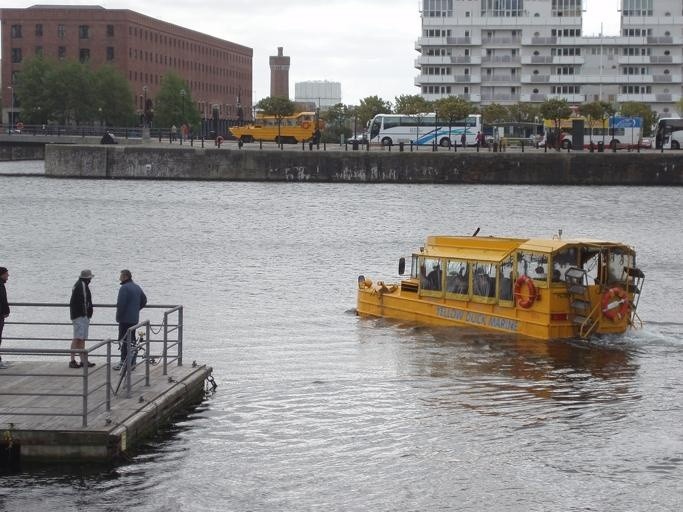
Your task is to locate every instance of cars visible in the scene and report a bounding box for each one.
[641,135,652,148]
[345,135,365,145]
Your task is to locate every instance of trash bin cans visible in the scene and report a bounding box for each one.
[597,141,604,152]
[493,143,497,152]
[352,140,358,150]
[399,142,403,151]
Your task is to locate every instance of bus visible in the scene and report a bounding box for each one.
[539,113,642,150]
[365,112,484,148]
[652,116,683,150]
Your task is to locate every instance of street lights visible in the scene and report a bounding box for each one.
[140,84,152,141]
[179,89,189,127]
[7,86,16,129]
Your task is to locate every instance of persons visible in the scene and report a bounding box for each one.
[423,263,562,300]
[67,268,96,369]
[474,131,483,147]
[111,268,147,370]
[0,266,10,369]
[460,133,465,148]
[170,124,177,141]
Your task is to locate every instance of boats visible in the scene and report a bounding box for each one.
[228,109,317,145]
[352,222,645,341]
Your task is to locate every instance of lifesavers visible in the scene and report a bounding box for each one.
[601,287,629,322]
[513,275,535,308]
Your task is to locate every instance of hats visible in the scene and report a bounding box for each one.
[0,267,8,275]
[79,269,94,279]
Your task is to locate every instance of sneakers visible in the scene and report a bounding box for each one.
[0,362,9,368]
[69,361,81,368]
[112,362,124,370]
[80,361,96,367]
